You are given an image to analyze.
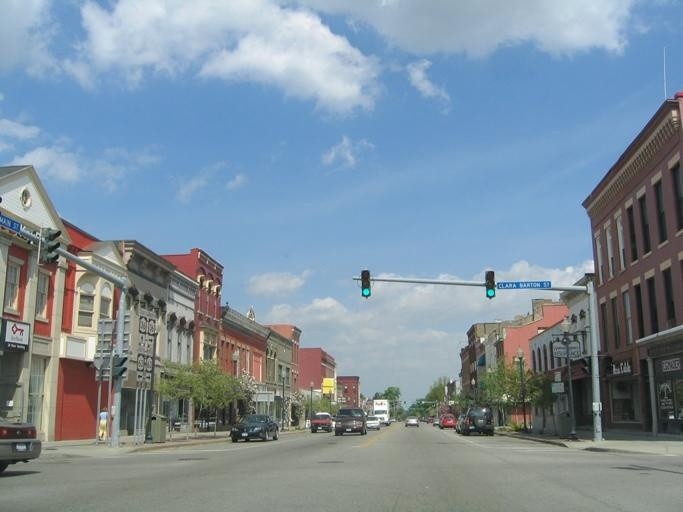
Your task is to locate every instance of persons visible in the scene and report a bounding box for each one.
[98,407,108,441]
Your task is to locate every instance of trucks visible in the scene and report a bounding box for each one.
[373,399,391,426]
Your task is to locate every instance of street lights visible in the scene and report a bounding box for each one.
[308,379,314,416]
[340,392,343,406]
[470,378,476,407]
[143,319,162,444]
[329,387,332,408]
[231,349,241,377]
[280,368,287,431]
[551,315,582,441]
[516,344,527,432]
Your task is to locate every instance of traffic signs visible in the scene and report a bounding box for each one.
[0,213,23,235]
[496,279,551,289]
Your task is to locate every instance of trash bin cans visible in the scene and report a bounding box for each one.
[557,411,572,439]
[151,414,168,443]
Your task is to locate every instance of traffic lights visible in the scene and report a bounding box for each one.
[112,354,127,377]
[360,269,371,298]
[484,271,495,298]
[41,227,61,264]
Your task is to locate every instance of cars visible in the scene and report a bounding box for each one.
[419,416,439,426]
[390,418,396,422]
[308,414,333,433]
[405,415,419,427]
[438,413,457,429]
[230,414,278,442]
[454,415,466,433]
[315,412,335,429]
[366,415,381,429]
[0,415,41,473]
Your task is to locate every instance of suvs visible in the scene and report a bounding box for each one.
[335,407,367,435]
[460,407,493,436]
